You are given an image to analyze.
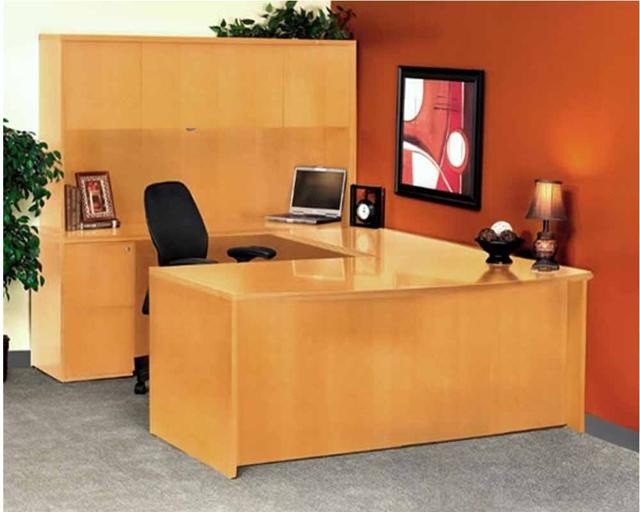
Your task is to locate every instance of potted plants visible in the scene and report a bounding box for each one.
[3,116,65,383]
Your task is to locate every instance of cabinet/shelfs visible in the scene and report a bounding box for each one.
[39,34,356,136]
[64,215,594,478]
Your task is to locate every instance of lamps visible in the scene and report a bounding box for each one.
[523,177,568,273]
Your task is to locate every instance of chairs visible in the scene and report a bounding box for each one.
[132,180,277,395]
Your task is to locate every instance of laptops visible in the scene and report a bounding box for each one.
[266,166,347,225]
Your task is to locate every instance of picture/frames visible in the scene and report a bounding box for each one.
[75,169,117,223]
[393,64,484,212]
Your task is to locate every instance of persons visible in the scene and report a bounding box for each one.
[85,180,104,214]
